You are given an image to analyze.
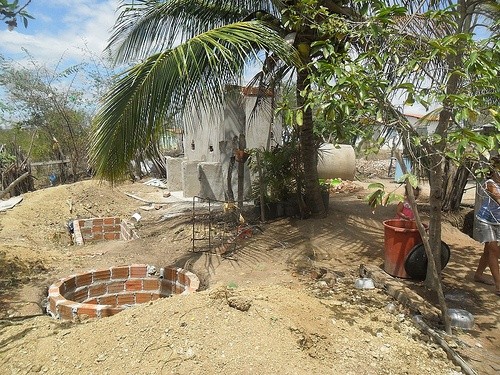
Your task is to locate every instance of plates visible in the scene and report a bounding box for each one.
[355,278,375,290]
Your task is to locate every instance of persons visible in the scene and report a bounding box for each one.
[395,185,419,219]
[474,156,500,296]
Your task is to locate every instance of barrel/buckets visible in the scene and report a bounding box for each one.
[253,188,330,220]
[394,158,412,183]
[383,219,429,279]
